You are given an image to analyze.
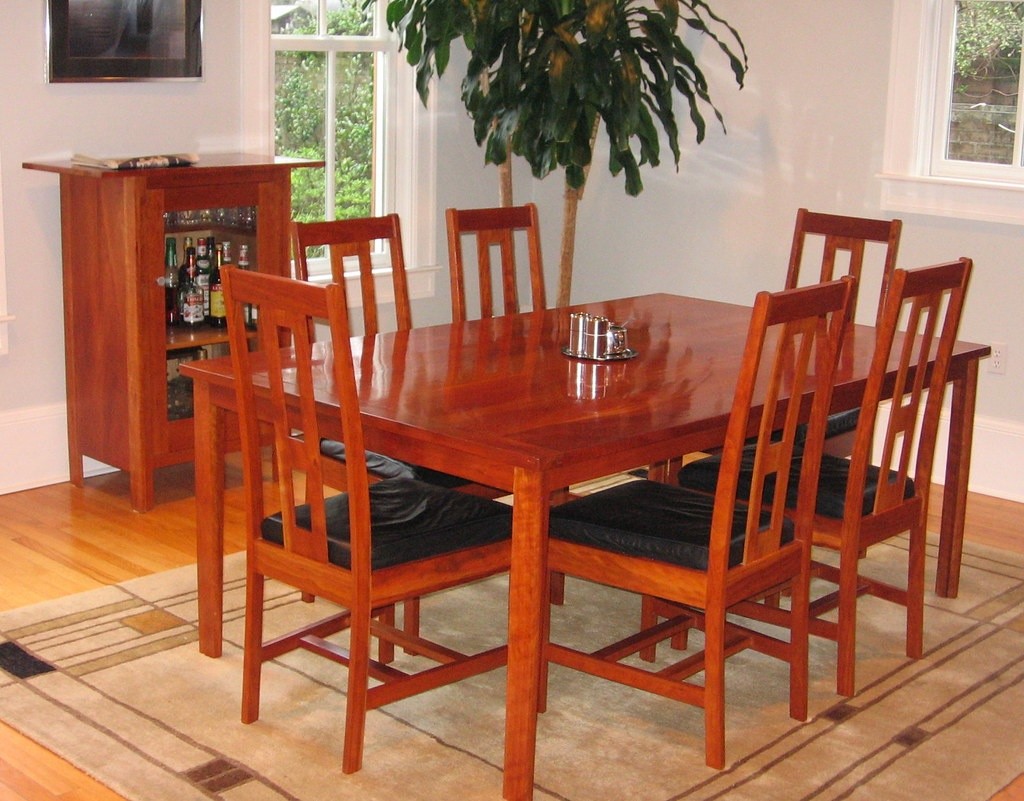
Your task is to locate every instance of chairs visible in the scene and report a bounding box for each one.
[679,207,903,460]
[289,212,510,498]
[536,275,853,769]
[670,257,972,702]
[218,264,513,775]
[445,203,546,323]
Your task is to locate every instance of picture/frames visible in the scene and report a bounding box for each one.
[48,0,204,83]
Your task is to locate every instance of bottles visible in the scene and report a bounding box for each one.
[569,312,589,355]
[195,349,207,360]
[164,236,260,330]
[585,316,608,359]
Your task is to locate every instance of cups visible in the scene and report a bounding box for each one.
[165,205,256,228]
[606,326,628,354]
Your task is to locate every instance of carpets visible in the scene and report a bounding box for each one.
[0,473,1024,801]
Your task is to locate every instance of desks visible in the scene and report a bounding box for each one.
[178,292,991,801]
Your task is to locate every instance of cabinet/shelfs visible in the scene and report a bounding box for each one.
[22,154,325,513]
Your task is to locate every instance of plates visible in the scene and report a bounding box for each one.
[561,344,638,360]
